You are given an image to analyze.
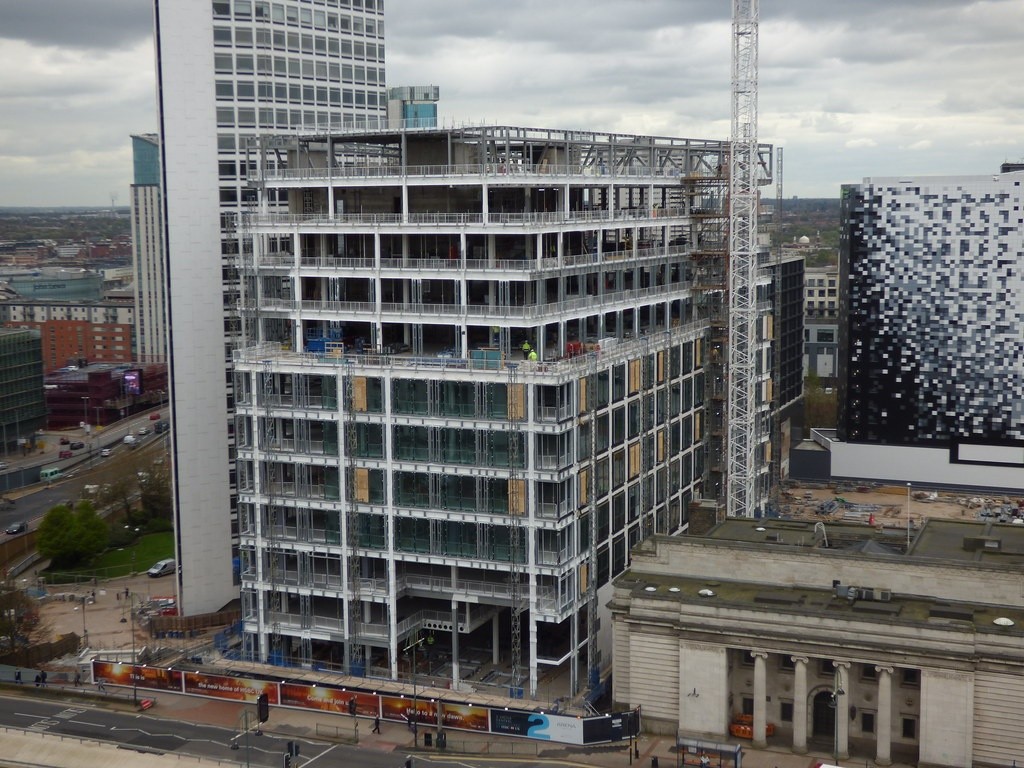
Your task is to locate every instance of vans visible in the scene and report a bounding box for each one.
[147,558,175,578]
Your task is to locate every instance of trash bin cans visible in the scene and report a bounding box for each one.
[424,734,432,745]
[436,732,446,748]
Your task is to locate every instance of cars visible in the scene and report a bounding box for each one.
[5,522,29,534]
[59,413,169,458]
[41,468,64,483]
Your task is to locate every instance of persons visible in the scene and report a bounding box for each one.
[125,588,129,599]
[15,667,23,684]
[528,349,537,360]
[523,340,530,360]
[74,669,83,687]
[98,676,105,691]
[91,591,96,603]
[700,751,709,765]
[568,342,587,358]
[372,714,381,734]
[36,670,47,687]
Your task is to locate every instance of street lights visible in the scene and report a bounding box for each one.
[907,483,911,548]
[830,669,844,765]
[81,397,89,447]
[231,709,262,768]
[120,593,146,708]
[93,406,102,464]
[74,594,95,650]
[157,389,166,459]
[403,627,426,750]
